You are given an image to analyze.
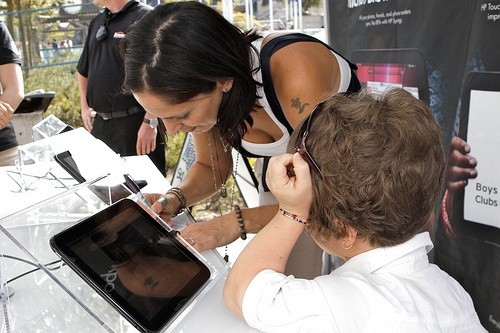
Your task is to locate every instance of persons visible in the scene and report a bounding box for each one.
[0,20,25,152]
[122,2,362,278]
[222,87,489,333]
[76,0,166,177]
[441,135,478,192]
[38,37,75,62]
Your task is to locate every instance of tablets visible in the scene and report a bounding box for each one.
[55,142,148,204]
[49,198,211,333]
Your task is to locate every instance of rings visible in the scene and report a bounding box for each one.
[156,196,169,208]
[190,237,194,244]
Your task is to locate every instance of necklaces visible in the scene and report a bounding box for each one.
[213,135,232,197]
[207,123,240,262]
[219,136,229,153]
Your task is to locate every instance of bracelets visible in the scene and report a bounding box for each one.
[235,205,247,240]
[280,207,308,227]
[167,188,186,217]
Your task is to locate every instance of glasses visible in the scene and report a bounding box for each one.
[294,101,322,179]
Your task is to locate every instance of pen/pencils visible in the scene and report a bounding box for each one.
[124,173,152,208]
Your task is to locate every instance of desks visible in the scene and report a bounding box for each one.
[0,155,255,332]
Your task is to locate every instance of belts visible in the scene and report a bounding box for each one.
[96,105,145,122]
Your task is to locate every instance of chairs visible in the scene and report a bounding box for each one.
[14,92,56,136]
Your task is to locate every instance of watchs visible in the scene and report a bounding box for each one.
[142,118,159,129]
